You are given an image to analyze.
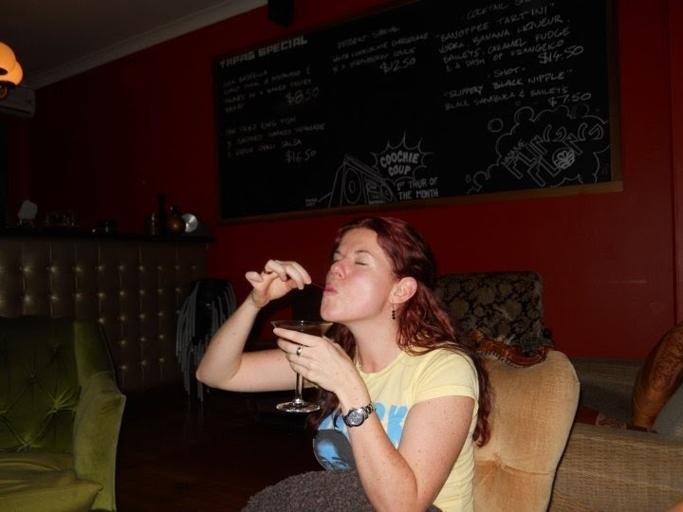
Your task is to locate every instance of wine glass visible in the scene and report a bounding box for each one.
[267,319,338,413]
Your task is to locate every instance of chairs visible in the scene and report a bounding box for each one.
[424,325,582,512]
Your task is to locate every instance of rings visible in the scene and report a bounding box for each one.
[296,344,304,356]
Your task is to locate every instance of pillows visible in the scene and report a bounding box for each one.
[624,321,680,430]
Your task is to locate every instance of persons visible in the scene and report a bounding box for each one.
[313,429,356,471]
[194,215,496,512]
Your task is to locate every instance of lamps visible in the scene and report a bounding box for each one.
[0,36,24,98]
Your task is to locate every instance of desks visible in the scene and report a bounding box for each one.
[1,223,216,414]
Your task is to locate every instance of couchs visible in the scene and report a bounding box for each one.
[435,268,544,350]
[2,308,130,512]
[549,306,681,512]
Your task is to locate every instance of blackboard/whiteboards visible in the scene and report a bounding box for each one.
[210,0,624,225]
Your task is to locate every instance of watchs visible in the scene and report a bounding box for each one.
[341,401,377,427]
